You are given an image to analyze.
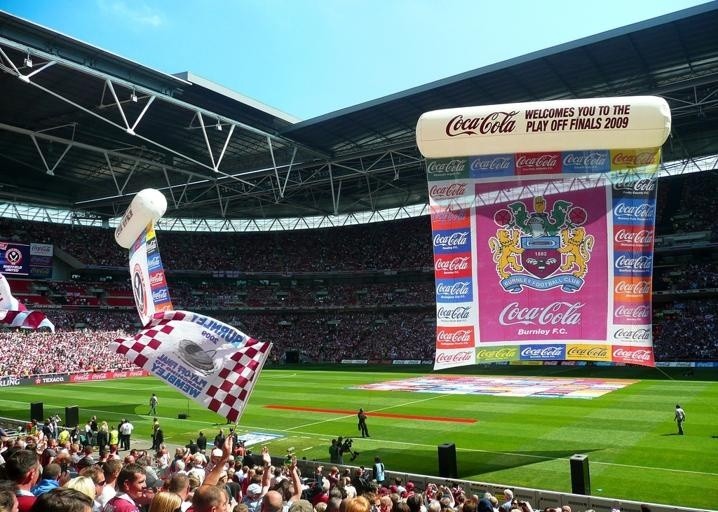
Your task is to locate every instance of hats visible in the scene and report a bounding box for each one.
[77,456,99,470]
[213,449,223,457]
[391,487,397,493]
[406,483,414,488]
[380,488,387,496]
[477,500,493,512]
[246,483,261,497]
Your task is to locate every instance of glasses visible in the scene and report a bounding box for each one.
[96,480,106,486]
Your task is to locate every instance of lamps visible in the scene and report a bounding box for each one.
[130,88,137,102]
[24,54,32,69]
[216,119,223,130]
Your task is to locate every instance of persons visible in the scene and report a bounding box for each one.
[358,408,371,437]
[146,393,159,416]
[156,215,437,365]
[652,170,717,362]
[0,415,652,512]
[0,216,142,376]
[673,404,685,434]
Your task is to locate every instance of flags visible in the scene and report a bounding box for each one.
[106,310,271,425]
